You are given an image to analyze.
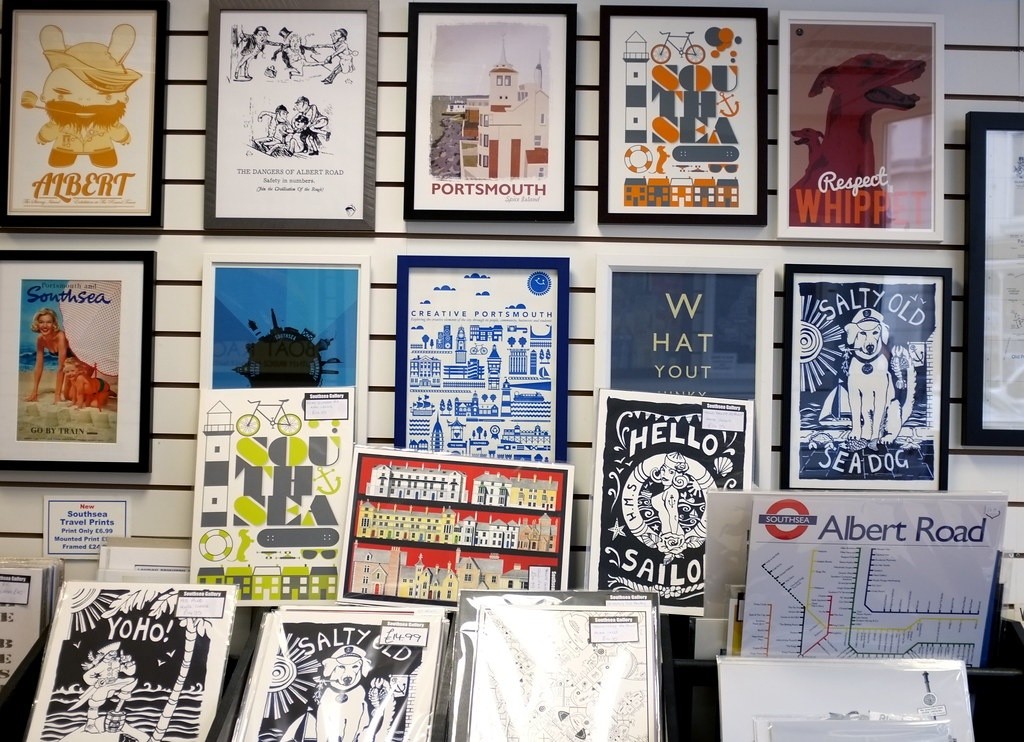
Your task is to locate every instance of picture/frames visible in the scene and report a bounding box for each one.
[336,444,575,606]
[402,3,577,223]
[596,255,775,487]
[779,262,953,490]
[0,0,169,233]
[0,250,156,473]
[200,256,370,441]
[202,0,380,232]
[596,6,769,226]
[391,257,573,458]
[775,9,944,240]
[959,112,1024,445]
